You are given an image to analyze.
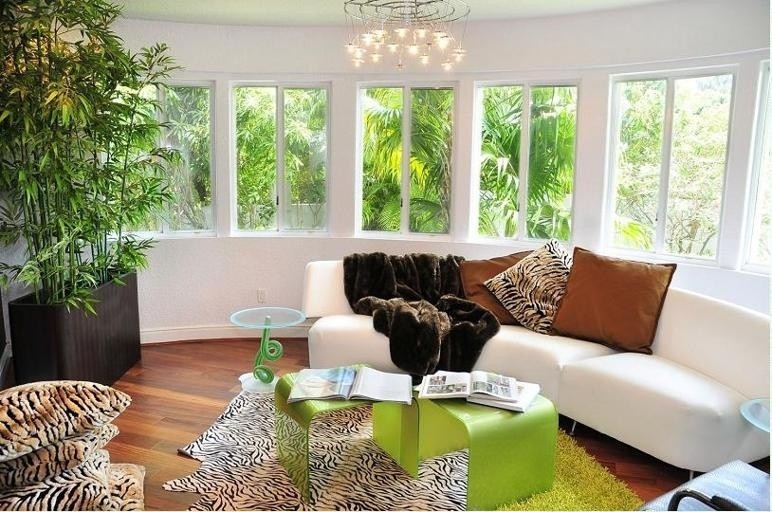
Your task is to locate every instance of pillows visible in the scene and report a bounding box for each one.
[458,237,677,355]
[0,379,146,512]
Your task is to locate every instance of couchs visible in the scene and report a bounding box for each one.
[300,259,770,480]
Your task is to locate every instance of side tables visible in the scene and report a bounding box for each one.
[739,398,770,433]
[229,306,306,393]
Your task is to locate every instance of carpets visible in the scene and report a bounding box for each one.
[493,427,645,511]
[161,390,470,511]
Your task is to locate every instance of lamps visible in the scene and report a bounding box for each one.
[343,1,471,71]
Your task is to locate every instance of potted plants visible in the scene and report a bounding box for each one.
[0,0,186,394]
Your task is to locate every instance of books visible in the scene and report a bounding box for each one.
[418,370,521,403]
[283,365,414,404]
[413,368,541,414]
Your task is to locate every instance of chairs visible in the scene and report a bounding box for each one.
[634,460,770,511]
